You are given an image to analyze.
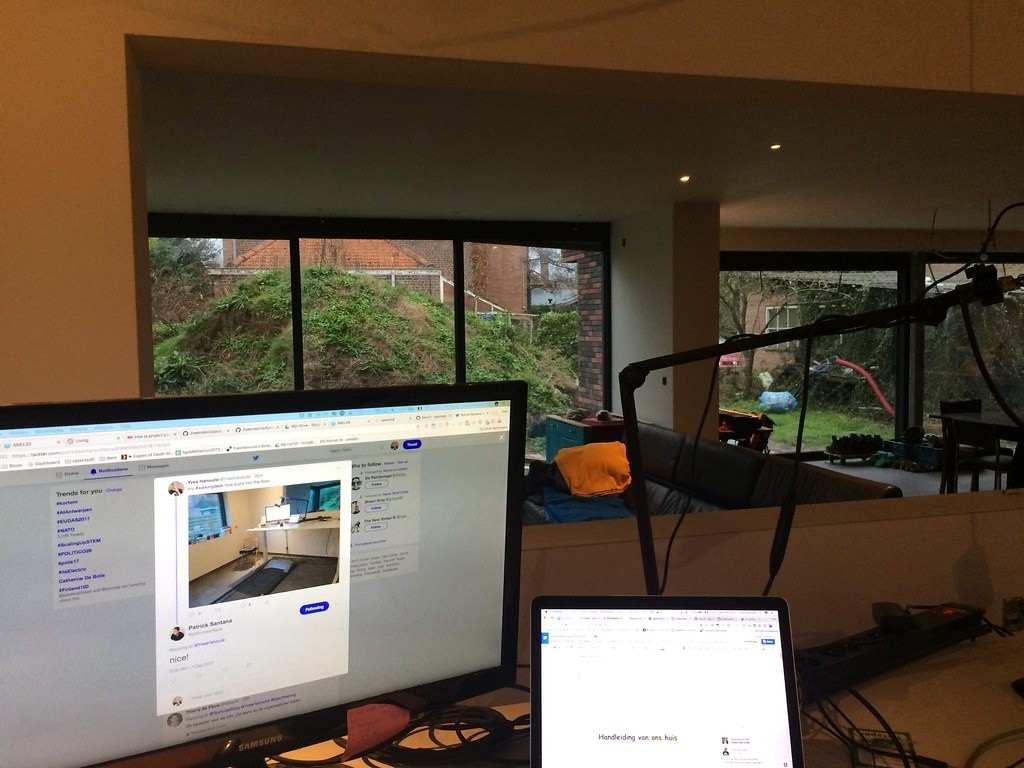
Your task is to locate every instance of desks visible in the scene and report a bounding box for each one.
[929,412,1024,494]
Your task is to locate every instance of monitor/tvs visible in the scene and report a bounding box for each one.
[0,381,529,768]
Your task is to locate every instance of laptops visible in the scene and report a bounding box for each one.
[530,595,805,768]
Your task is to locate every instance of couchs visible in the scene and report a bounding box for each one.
[523,422,830,524]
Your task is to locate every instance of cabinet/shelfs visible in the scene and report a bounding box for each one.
[545,409,624,462]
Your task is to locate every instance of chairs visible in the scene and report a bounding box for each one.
[939,398,1014,495]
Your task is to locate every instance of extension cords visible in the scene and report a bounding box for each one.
[795,603,984,705]
